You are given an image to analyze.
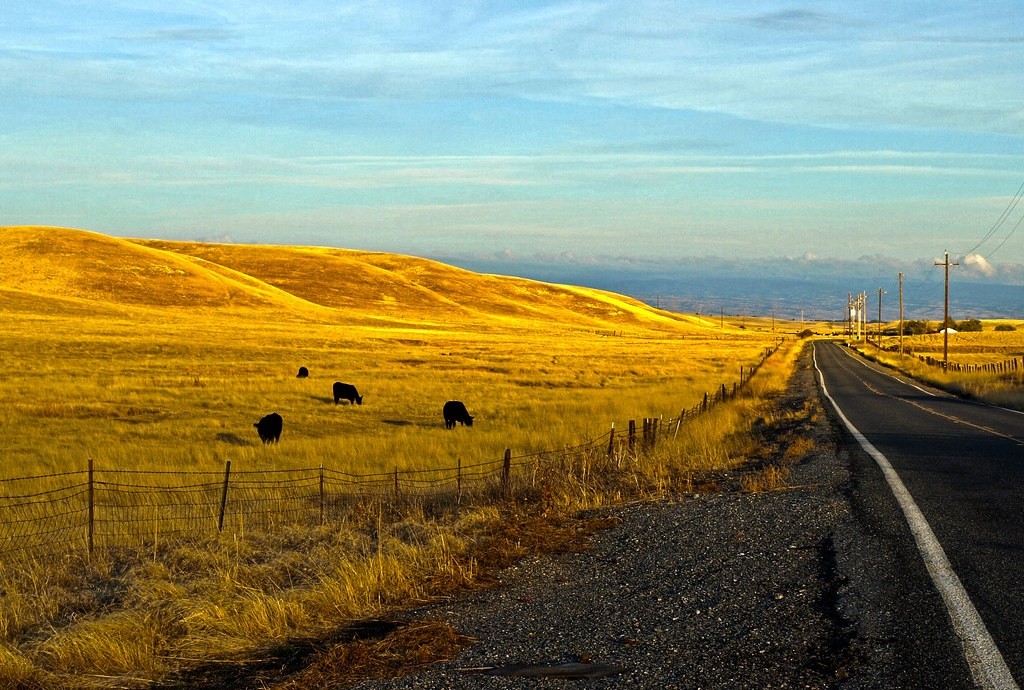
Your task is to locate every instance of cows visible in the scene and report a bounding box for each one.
[297,367,308,378]
[253,412,284,446]
[443,401,475,430]
[333,382,364,406]
